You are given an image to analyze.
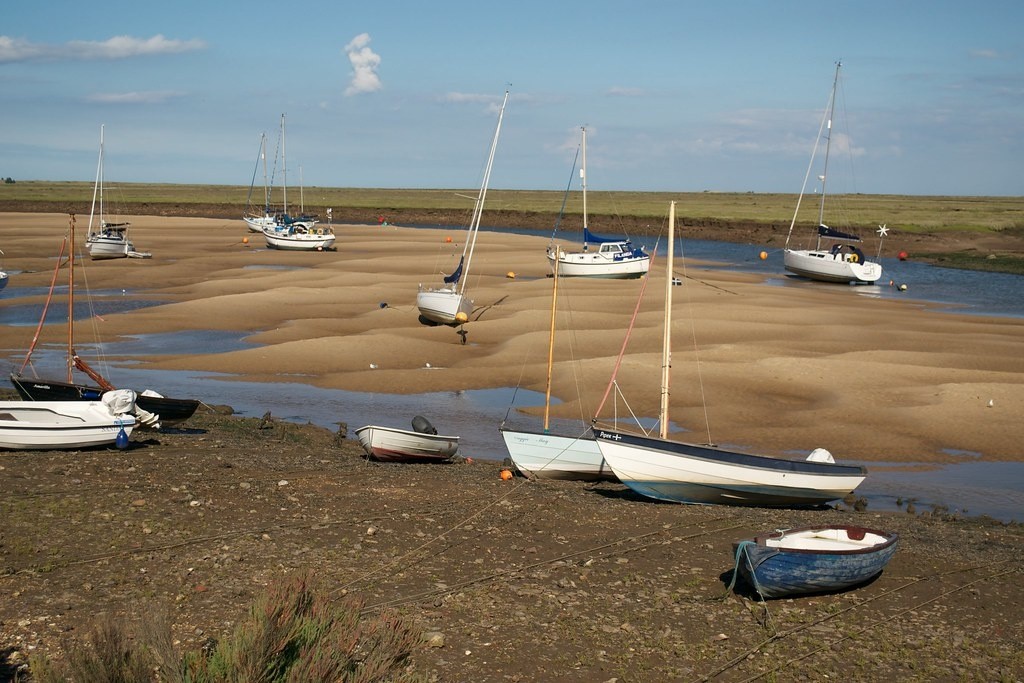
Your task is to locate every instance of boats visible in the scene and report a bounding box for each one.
[734,525,901,602]
[547,123,648,279]
[10,202,201,422]
[263,113,336,251]
[782,59,883,283]
[85,124,135,259]
[0,399,136,450]
[498,245,617,481]
[355,414,460,461]
[589,202,868,506]
[416,90,512,323]
[242,132,321,231]
